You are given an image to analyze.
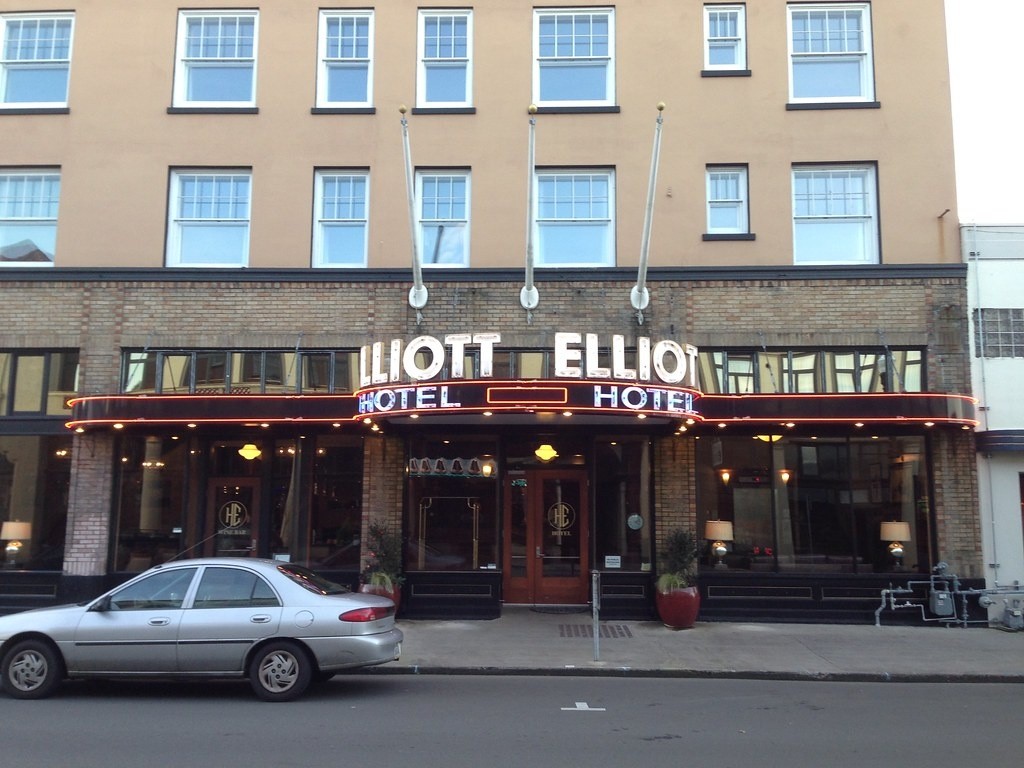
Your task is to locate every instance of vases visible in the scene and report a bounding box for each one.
[359,582,401,612]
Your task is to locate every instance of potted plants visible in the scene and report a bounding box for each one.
[655,528,700,630]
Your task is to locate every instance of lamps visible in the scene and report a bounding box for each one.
[534,437,557,460]
[703,519,734,570]
[880,519,912,572]
[238,440,262,460]
[483,458,497,477]
[0,519,32,569]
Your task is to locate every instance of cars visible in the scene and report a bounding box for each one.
[318,538,471,572]
[0,557,404,702]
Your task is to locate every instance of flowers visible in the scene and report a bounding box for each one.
[359,518,406,594]
